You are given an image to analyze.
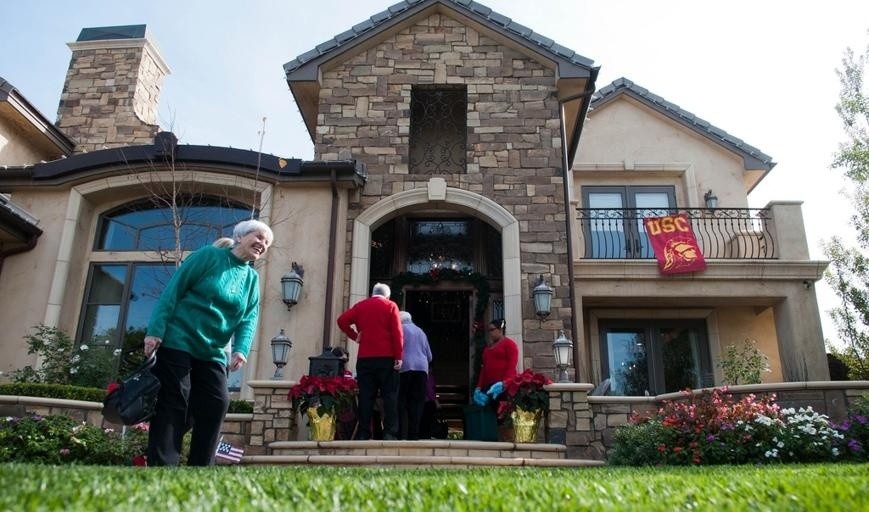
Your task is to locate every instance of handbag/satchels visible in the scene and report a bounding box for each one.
[101,348,164,427]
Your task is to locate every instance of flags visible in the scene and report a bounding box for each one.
[216,441,245,465]
[643,210,711,278]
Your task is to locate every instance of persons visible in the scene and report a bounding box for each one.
[132,235,237,467]
[142,218,277,468]
[371,387,387,440]
[335,279,405,441]
[418,364,442,441]
[474,318,521,442]
[393,309,434,442]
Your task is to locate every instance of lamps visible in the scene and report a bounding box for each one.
[703,189,717,214]
[269,327,293,380]
[278,260,306,312]
[551,328,574,383]
[533,272,553,322]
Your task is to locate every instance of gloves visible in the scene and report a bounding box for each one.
[474,381,503,408]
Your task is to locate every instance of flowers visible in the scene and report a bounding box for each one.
[494,366,553,431]
[284,373,359,426]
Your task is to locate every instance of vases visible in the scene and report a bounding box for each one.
[510,406,543,443]
[306,406,338,441]
